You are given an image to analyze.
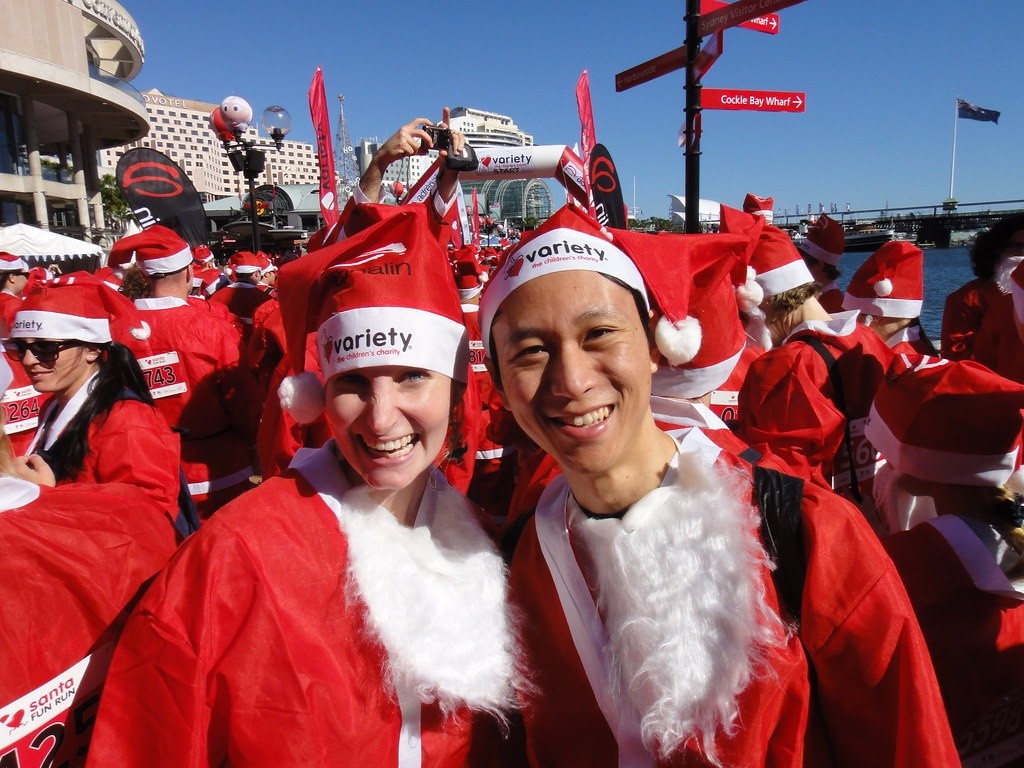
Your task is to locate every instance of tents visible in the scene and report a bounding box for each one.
[0,223,105,275]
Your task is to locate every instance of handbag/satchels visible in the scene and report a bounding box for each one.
[837,486,888,540]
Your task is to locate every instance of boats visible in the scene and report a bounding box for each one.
[786,220,916,251]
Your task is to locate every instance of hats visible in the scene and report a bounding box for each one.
[642,233,749,402]
[191,244,214,263]
[256,250,278,276]
[21,267,53,301]
[223,250,262,276]
[0,252,30,273]
[718,203,816,314]
[190,262,204,288]
[743,193,774,225]
[193,268,228,296]
[1010,257,1024,325]
[106,223,193,276]
[475,200,703,413]
[94,267,124,292]
[796,212,845,268]
[863,351,1023,486]
[10,270,150,344]
[444,239,514,300]
[841,240,924,319]
[278,198,469,425]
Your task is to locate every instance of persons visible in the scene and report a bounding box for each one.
[479,203,961,768]
[0,106,1024,539]
[864,348,1024,768]
[81,202,530,768]
[0,353,186,768]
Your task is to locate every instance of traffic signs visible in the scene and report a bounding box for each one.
[700,86,807,112]
[699,0,806,34]
[678,114,701,148]
[615,46,686,93]
[694,27,724,83]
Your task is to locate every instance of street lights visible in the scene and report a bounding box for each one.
[209,96,292,250]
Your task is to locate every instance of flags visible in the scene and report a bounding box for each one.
[957,97,1001,125]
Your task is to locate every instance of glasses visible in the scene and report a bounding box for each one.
[1,337,79,363]
